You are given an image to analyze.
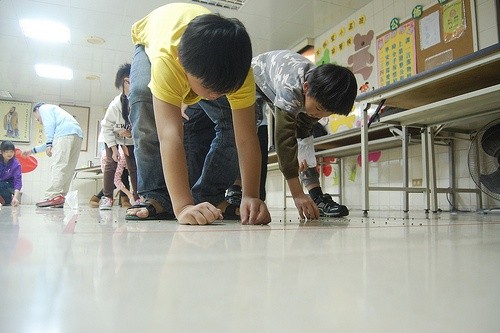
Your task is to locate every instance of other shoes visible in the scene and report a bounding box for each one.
[36,194,64,208]
[99,196,112,210]
[121,196,132,209]
[89,195,100,207]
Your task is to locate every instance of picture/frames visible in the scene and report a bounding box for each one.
[59,104,90,152]
[0,99,34,143]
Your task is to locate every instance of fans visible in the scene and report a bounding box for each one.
[467,117,500,214]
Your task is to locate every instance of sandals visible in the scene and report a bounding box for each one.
[125,198,175,221]
[219,201,249,221]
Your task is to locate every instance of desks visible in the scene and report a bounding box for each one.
[74,165,103,195]
[266,40,500,214]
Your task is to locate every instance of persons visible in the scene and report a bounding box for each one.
[88,63,242,209]
[0,140,23,207]
[129,3,271,226]
[20,103,83,208]
[251,50,357,220]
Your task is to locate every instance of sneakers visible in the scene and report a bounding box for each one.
[226,188,242,204]
[313,193,349,217]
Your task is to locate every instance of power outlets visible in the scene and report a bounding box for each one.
[412,178,422,187]
